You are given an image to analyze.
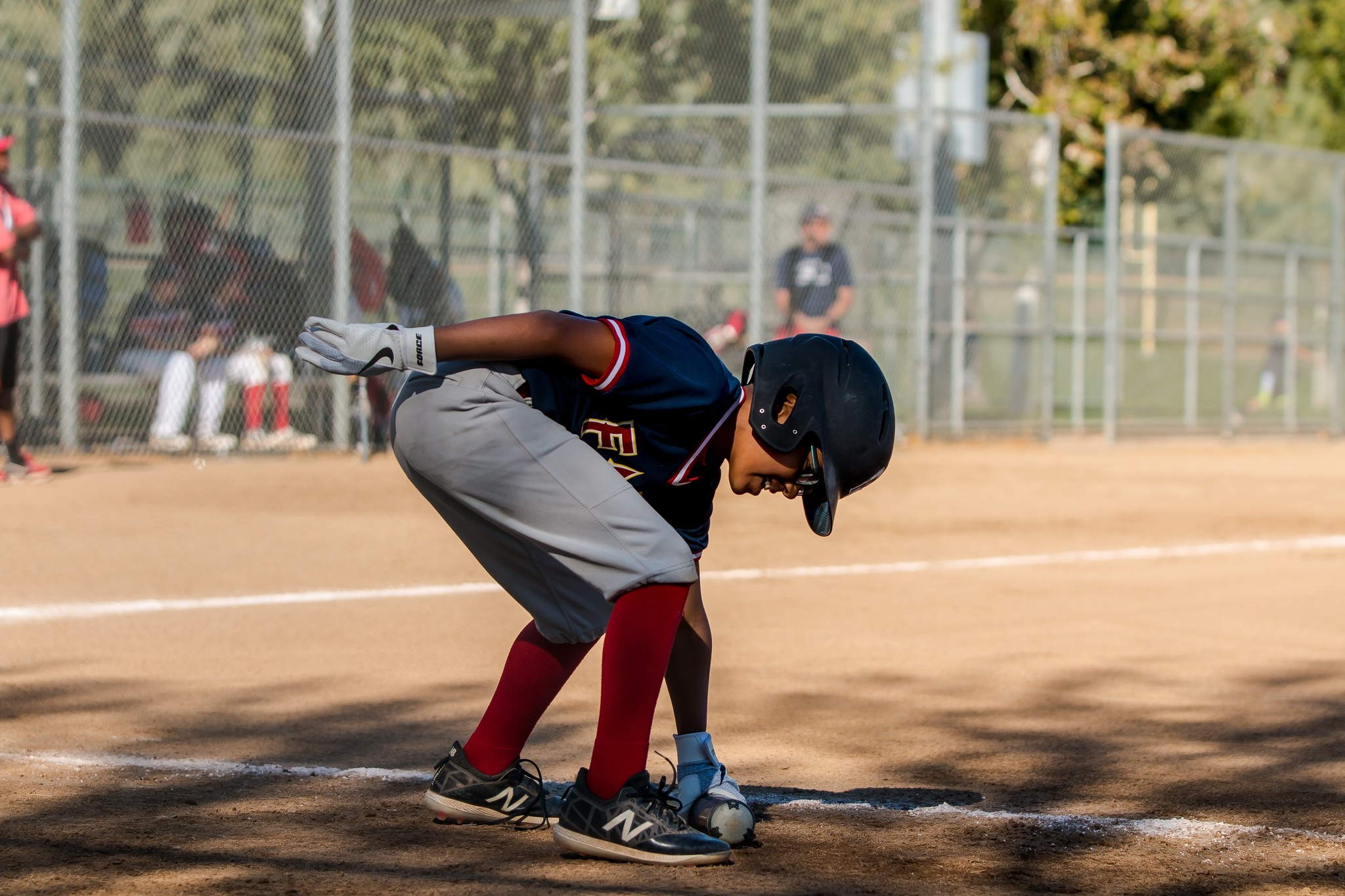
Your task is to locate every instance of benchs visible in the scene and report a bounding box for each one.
[19,372,337,458]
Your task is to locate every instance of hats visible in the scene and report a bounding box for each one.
[798,202,834,223]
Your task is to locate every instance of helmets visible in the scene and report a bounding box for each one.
[740,333,894,537]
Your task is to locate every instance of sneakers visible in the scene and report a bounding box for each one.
[421,740,574,826]
[552,766,730,864]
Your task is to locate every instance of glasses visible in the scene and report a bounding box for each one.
[796,445,820,496]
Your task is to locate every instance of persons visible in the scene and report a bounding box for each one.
[348,216,466,329]
[1244,316,1312,410]
[771,205,855,336]
[82,172,318,452]
[295,310,896,864]
[0,128,52,487]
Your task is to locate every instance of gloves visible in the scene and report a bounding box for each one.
[296,316,437,377]
[672,732,746,821]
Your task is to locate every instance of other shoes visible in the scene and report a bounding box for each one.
[8,450,51,480]
[148,432,194,453]
[268,422,318,451]
[239,429,280,452]
[190,433,239,451]
